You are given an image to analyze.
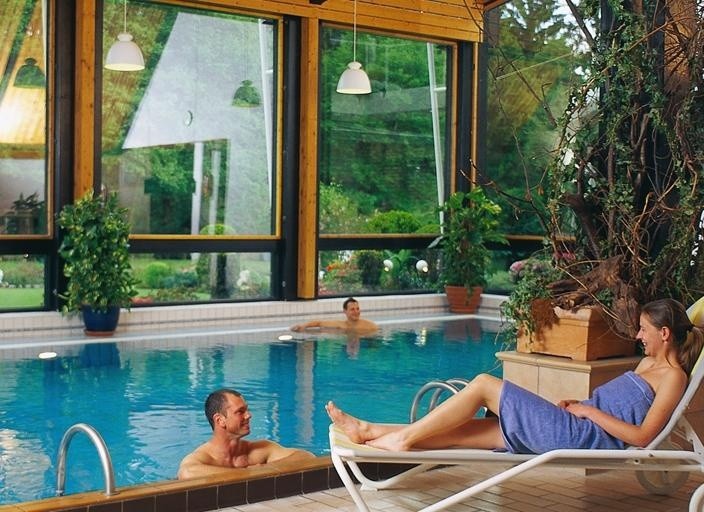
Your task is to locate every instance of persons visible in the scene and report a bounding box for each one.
[342,336,361,358]
[322,296,704,460]
[289,296,382,334]
[175,387,318,479]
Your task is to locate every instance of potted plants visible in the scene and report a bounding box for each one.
[427,182,513,314]
[50,182,143,338]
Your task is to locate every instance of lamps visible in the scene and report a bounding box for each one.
[102,0,147,76]
[11,58,45,91]
[336,0,373,98]
[230,77,264,107]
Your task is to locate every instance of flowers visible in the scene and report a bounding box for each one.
[494,251,584,359]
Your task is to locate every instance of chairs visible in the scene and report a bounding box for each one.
[328,291,703,512]
